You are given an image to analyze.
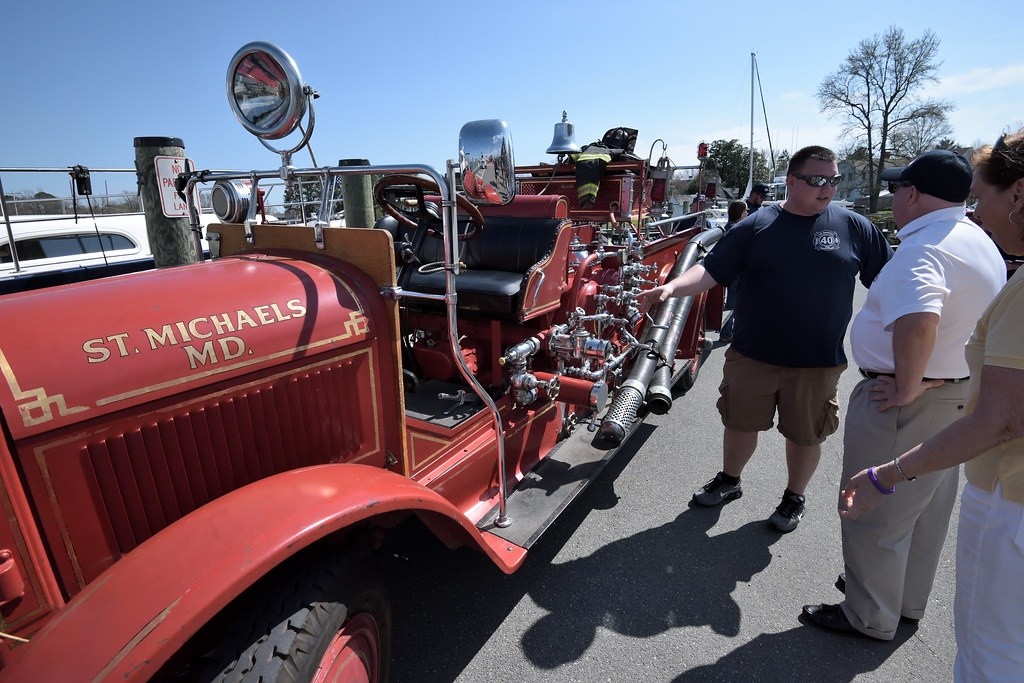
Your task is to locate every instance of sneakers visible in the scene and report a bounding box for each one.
[768,489,806,532]
[693,471,743,505]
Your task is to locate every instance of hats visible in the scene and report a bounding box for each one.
[881,150,974,202]
[752,184,773,197]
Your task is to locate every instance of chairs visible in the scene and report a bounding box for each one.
[366,206,578,317]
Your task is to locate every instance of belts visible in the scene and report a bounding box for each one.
[859,367,971,382]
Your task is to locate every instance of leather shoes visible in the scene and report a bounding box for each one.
[803,603,890,642]
[838,573,919,624]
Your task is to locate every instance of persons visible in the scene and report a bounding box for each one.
[802,151,1007,643]
[632,145,895,533]
[718,202,750,342]
[838,130,1024,683]
[746,184,772,217]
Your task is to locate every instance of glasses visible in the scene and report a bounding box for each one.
[993,133,1024,165]
[745,208,750,213]
[887,181,912,194]
[792,173,842,187]
[758,194,766,199]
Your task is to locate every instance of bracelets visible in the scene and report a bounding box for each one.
[895,458,917,483]
[867,466,896,495]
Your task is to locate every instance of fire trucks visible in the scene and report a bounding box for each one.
[2,41,736,683]
